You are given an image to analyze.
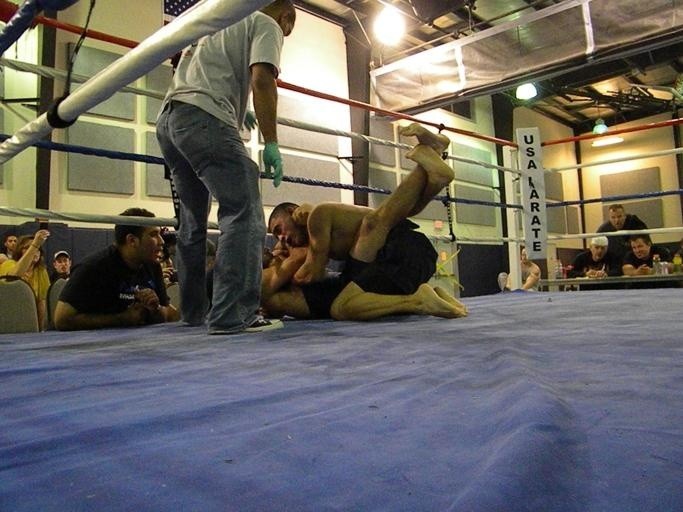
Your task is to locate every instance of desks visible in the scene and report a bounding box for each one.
[537,271,683,291]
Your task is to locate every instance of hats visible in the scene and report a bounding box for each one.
[53,249,73,262]
[591,235,608,247]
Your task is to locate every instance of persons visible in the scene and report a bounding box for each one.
[266,201,470,321]
[0,207,270,332]
[621,234,672,288]
[566,237,621,291]
[260,121,469,320]
[595,204,650,268]
[502,247,541,293]
[154,1,283,335]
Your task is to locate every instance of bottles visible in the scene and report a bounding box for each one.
[653,256,661,276]
[673,254,682,274]
[554,259,563,279]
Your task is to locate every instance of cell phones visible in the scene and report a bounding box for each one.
[39,222,49,230]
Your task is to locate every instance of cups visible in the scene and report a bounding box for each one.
[662,262,668,275]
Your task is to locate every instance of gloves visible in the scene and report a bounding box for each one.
[263,142,283,187]
[241,111,258,131]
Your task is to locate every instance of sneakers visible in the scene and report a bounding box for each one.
[246,319,285,333]
